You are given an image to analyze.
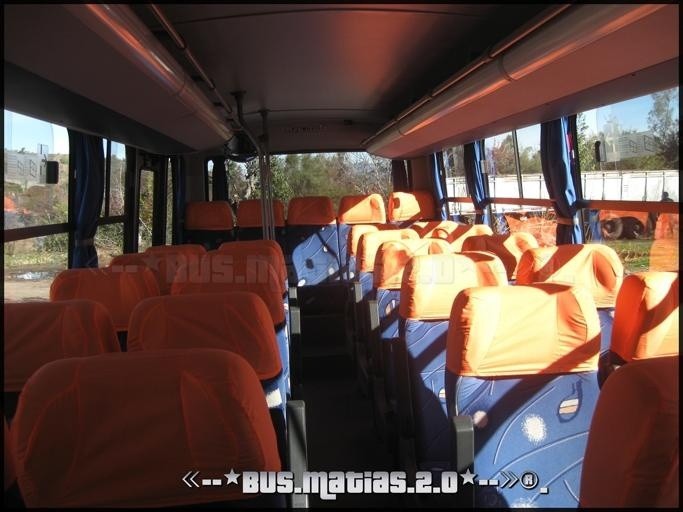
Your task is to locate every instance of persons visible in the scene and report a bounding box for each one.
[659,191,673,202]
